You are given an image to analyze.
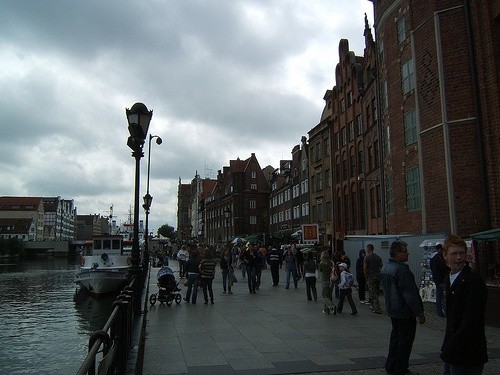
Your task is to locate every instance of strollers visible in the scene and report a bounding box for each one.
[148,266,185,306]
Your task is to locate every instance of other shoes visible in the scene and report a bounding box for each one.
[332,305,336,315]
[438,311,447,317]
[322,310,330,315]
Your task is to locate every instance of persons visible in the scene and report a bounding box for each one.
[356,249,368,303]
[165,275,181,292]
[152,246,177,267]
[334,251,348,297]
[383,241,426,375]
[220,244,303,294]
[440,235,488,375]
[178,245,217,303]
[303,251,317,301]
[364,244,383,313]
[320,251,337,315]
[430,251,449,315]
[337,263,358,315]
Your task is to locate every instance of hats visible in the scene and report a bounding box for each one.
[272,244,278,247]
[338,263,348,268]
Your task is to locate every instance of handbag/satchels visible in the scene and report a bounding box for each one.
[295,270,300,281]
[373,253,383,281]
[329,260,339,287]
[228,272,238,287]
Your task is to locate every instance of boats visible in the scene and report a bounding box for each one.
[74,250,132,298]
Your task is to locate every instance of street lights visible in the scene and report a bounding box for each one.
[142,133,163,267]
[125,101,154,278]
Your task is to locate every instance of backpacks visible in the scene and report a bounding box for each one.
[343,272,354,287]
[287,257,294,272]
